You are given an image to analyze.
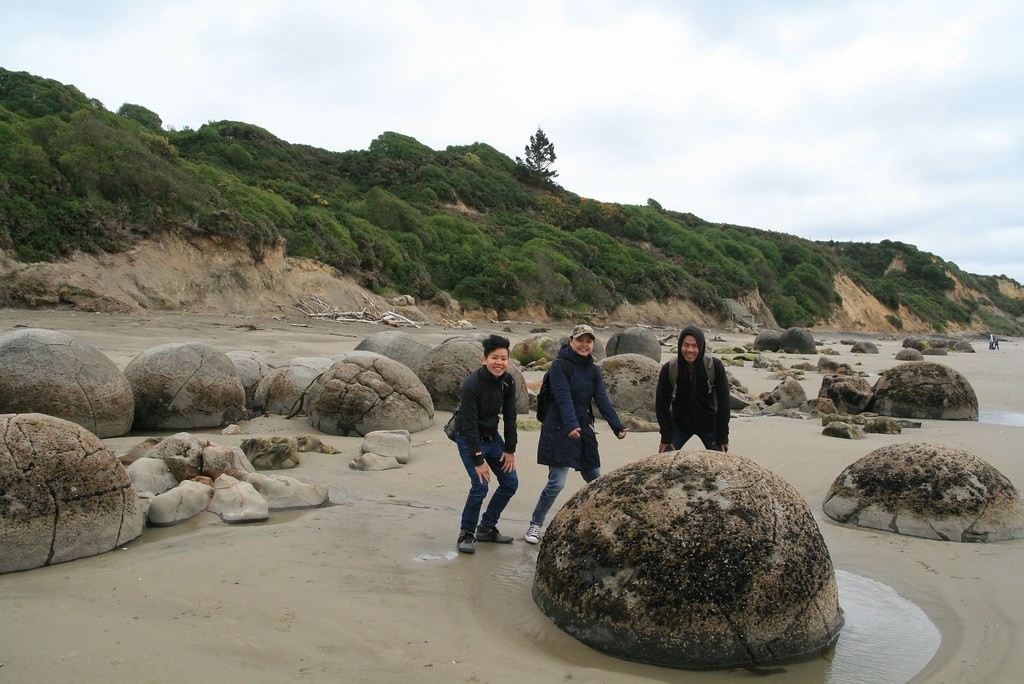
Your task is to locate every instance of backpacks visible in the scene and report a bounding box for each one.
[537,356,599,422]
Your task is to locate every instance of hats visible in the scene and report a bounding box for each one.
[569,324,596,343]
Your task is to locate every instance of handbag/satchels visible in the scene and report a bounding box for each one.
[443,407,459,442]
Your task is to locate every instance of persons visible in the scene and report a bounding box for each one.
[655,324,731,452]
[525,324,629,544]
[988,333,1000,350]
[455,335,519,553]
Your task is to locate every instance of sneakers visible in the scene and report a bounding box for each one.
[525,523,542,543]
[476,524,514,543]
[457,528,476,553]
